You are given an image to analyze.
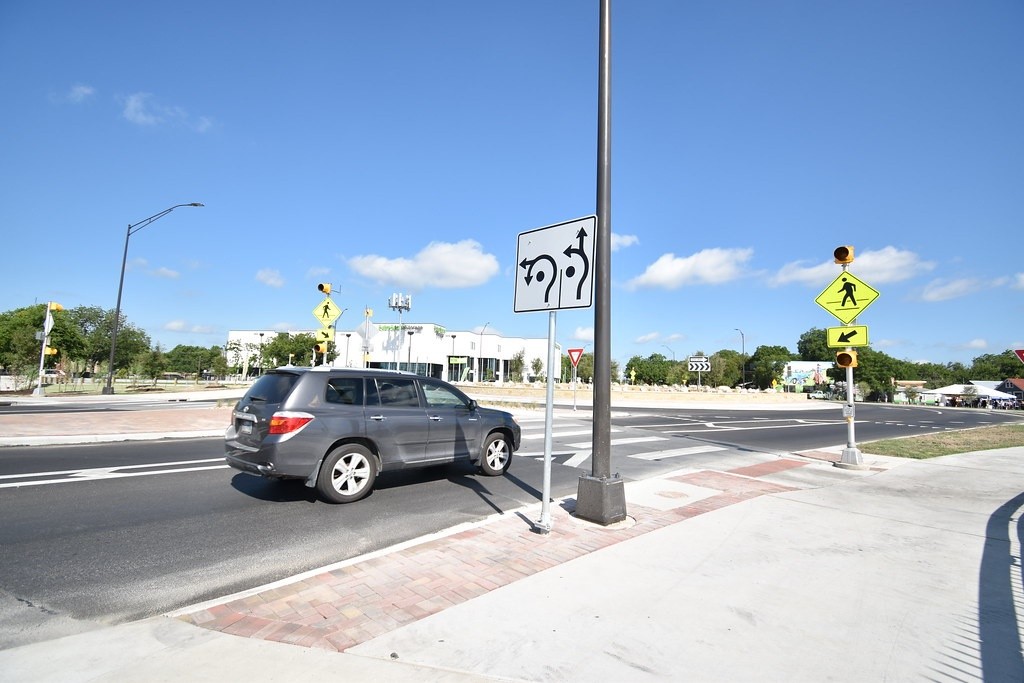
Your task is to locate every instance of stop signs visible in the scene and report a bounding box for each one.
[568,349,583,368]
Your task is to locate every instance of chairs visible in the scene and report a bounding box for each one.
[395,386,416,406]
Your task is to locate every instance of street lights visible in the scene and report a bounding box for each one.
[101,203,204,395]
[258,333,264,376]
[332,308,348,367]
[452,334,456,380]
[735,328,745,388]
[408,331,414,372]
[480,321,490,382]
[346,334,351,367]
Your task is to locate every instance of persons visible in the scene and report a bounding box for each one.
[380,384,396,402]
[950,396,1024,409]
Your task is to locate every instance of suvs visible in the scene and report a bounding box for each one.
[225,367,521,503]
[42,369,65,377]
[162,372,184,380]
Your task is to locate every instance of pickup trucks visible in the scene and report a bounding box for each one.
[808,390,827,399]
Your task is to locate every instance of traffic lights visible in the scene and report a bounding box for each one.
[836,351,858,368]
[834,245,854,264]
[48,302,62,312]
[318,283,330,294]
[313,344,325,353]
[45,347,57,356]
